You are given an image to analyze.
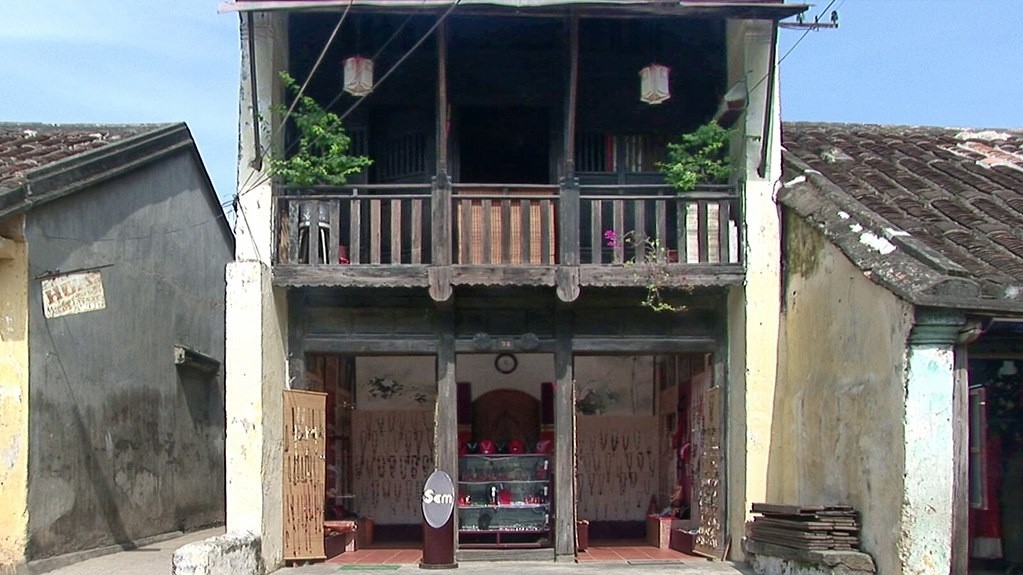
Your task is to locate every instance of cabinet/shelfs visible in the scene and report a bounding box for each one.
[458,454,554,549]
[296,516,374,562]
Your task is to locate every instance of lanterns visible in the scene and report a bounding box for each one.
[342,54,373,97]
[638,64,671,104]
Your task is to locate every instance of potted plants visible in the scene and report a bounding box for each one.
[266,69,374,224]
[652,117,745,263]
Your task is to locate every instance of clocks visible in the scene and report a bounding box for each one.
[495,353,518,375]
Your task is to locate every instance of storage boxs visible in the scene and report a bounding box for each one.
[645,517,698,556]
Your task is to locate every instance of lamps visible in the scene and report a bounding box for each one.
[342,12,374,96]
[640,18,671,104]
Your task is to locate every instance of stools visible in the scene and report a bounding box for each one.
[297,220,330,265]
